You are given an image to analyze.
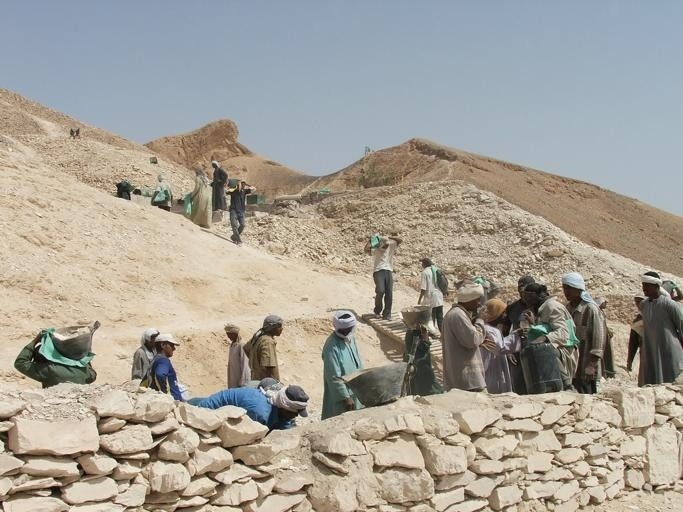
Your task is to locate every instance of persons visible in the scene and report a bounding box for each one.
[72,128,80,140]
[416,257,449,331]
[225,325,250,389]
[188,377,309,430]
[224,178,256,245]
[116,179,132,201]
[243,316,284,382]
[189,166,212,230]
[12,328,97,390]
[628,271,683,386]
[441,272,615,395]
[209,161,228,212]
[321,312,364,420]
[404,326,434,397]
[68,129,75,140]
[363,235,404,322]
[150,174,173,212]
[129,329,159,380]
[139,333,184,400]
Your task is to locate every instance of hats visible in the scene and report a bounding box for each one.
[285,385,308,418]
[257,377,282,393]
[154,333,181,346]
